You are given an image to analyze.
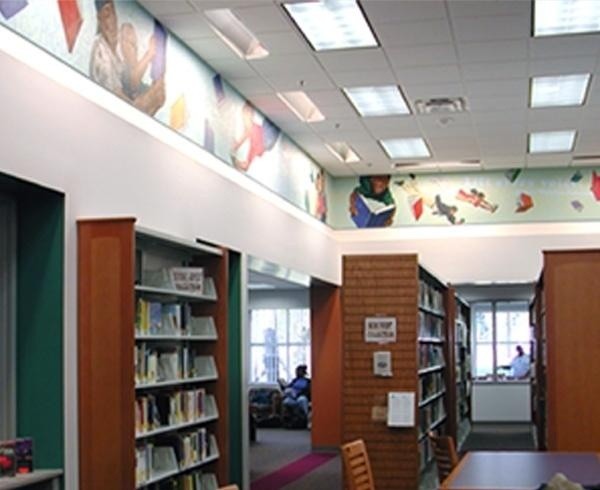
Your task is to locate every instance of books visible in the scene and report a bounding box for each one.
[133,266,221,490]
[410,195,423,221]
[572,201,584,212]
[149,17,167,80]
[350,191,396,228]
[529,285,546,452]
[416,279,472,490]
[514,192,535,213]
[0,0,29,20]
[569,169,583,183]
[211,72,226,104]
[56,0,84,52]
[203,119,215,154]
[169,93,189,130]
[504,167,522,183]
[590,169,600,202]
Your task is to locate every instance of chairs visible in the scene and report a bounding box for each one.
[338,436,374,489]
[427,429,463,486]
[248,384,284,428]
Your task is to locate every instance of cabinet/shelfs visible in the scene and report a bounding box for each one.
[341,252,450,490]
[528,248,600,453]
[448,281,475,452]
[76,215,231,490]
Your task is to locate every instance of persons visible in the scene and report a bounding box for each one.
[88,0,165,116]
[320,168,327,223]
[277,364,311,430]
[472,192,499,214]
[314,173,328,219]
[119,21,157,99]
[393,172,465,225]
[455,187,478,204]
[348,174,397,225]
[232,100,281,171]
[509,344,531,379]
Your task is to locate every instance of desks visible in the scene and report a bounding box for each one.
[468,377,532,424]
[1,468,63,490]
[437,450,600,490]
